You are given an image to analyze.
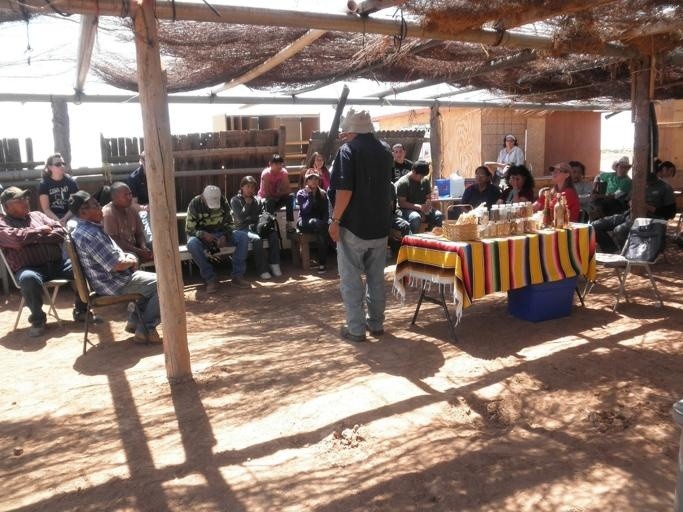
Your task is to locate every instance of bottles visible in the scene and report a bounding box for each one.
[480,201,536,238]
[433,185,439,199]
[543,191,569,230]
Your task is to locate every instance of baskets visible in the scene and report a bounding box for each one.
[442,204,479,241]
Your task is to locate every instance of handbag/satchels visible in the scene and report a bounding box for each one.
[625,222,666,261]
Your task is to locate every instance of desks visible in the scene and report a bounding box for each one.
[432,198,466,221]
[392,221,597,344]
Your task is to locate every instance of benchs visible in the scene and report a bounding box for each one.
[287,229,323,270]
[140,212,281,277]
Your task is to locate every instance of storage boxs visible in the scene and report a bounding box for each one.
[509,276,577,322]
[435,180,450,196]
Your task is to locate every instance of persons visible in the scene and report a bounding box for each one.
[0,151,163,345]
[327,109,394,342]
[460,133,676,253]
[185,140,445,294]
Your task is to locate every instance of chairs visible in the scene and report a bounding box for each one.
[65,232,149,355]
[1,246,72,330]
[581,216,667,319]
